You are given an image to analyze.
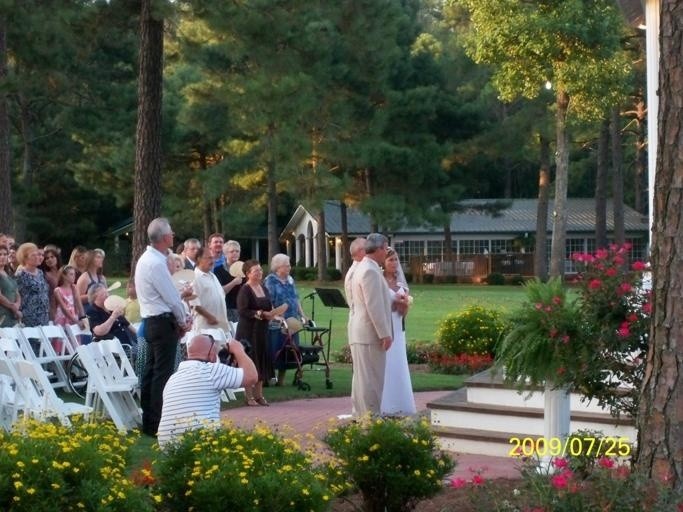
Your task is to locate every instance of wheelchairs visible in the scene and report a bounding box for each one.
[65,331,133,402]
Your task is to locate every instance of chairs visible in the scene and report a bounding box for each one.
[0,319,93,439]
[76,336,143,435]
[181,322,246,402]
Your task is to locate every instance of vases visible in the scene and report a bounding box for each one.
[534,379,572,475]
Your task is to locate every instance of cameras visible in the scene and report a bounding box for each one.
[217,338,253,366]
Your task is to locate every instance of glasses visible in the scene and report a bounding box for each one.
[170,232,176,238]
[253,268,264,272]
[202,333,215,356]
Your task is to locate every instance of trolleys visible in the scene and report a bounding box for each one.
[268,318,332,393]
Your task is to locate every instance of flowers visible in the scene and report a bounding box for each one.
[492,273,606,406]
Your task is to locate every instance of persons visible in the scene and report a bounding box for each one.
[134,217,193,437]
[352,233,393,418]
[214,240,247,324]
[383,247,416,417]
[124,282,142,325]
[14,243,50,327]
[344,237,367,424]
[68,245,88,285]
[236,259,277,407]
[157,334,258,457]
[77,248,108,314]
[189,247,231,331]
[207,232,226,273]
[176,238,202,270]
[87,282,137,377]
[0,230,22,329]
[263,254,310,387]
[45,249,61,322]
[51,264,87,355]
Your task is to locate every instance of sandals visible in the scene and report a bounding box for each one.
[255,396,269,406]
[246,396,260,407]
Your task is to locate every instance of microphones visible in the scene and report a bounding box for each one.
[303,290,317,299]
